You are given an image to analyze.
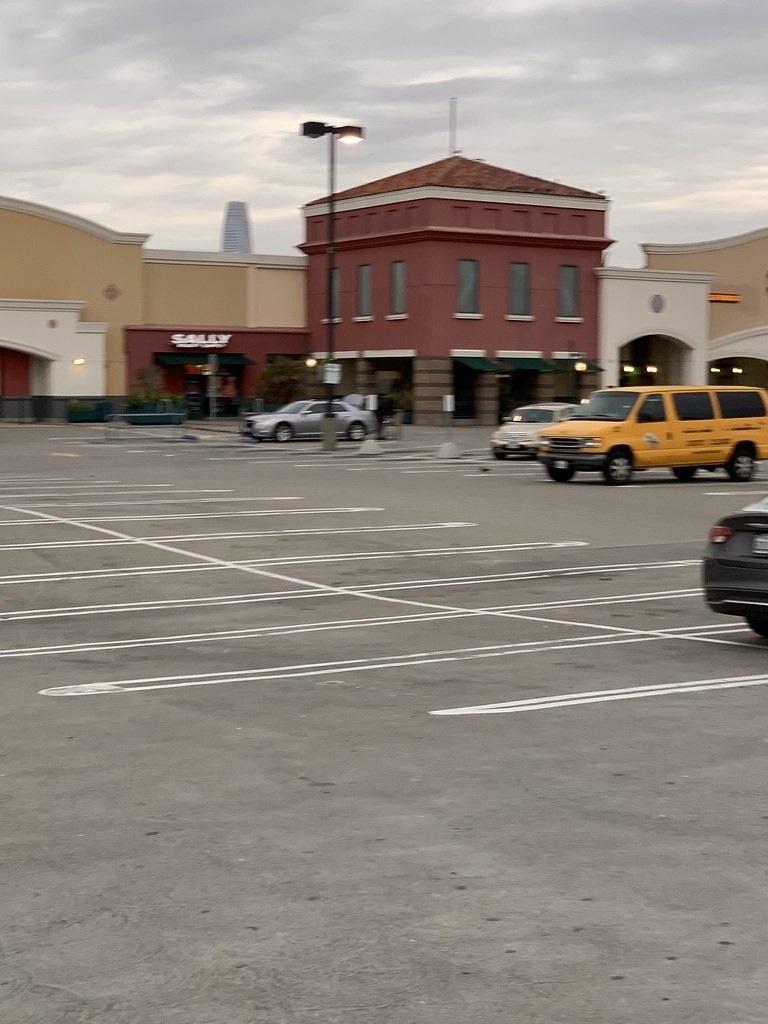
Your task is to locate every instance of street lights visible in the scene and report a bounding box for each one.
[301,121,363,418]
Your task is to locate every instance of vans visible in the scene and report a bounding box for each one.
[537,387,768,485]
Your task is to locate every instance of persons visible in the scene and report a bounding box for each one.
[362,387,386,441]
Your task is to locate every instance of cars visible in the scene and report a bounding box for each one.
[242,399,380,441]
[490,403,579,460]
[701,493,768,636]
